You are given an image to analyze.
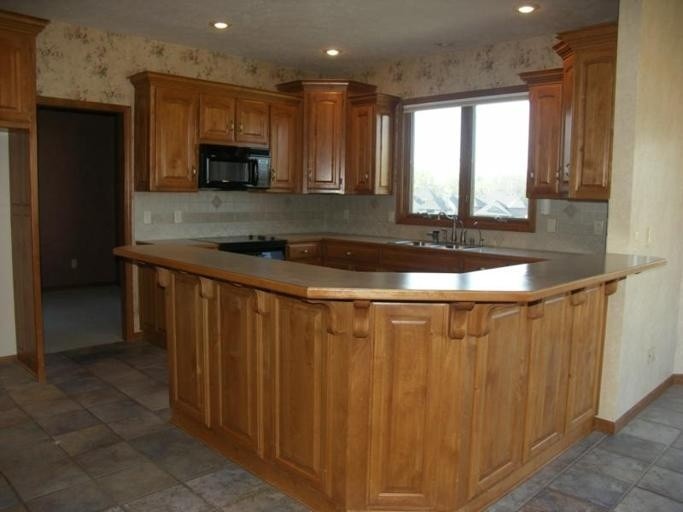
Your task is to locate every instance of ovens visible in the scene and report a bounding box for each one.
[217,246,285,261]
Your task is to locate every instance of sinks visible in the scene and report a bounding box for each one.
[388,239,496,249]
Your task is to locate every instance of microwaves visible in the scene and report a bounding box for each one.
[197,144,271,192]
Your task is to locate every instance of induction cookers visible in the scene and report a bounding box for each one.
[186,234,287,246]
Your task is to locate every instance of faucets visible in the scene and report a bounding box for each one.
[437,211,457,241]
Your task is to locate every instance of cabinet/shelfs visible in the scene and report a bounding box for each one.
[518,67,561,200]
[1,8,50,131]
[276,79,376,196]
[127,69,199,193]
[250,91,303,195]
[554,21,619,202]
[137,263,169,348]
[462,255,528,273]
[323,242,382,272]
[382,249,462,273]
[199,79,271,151]
[348,92,400,196]
[286,243,322,266]
